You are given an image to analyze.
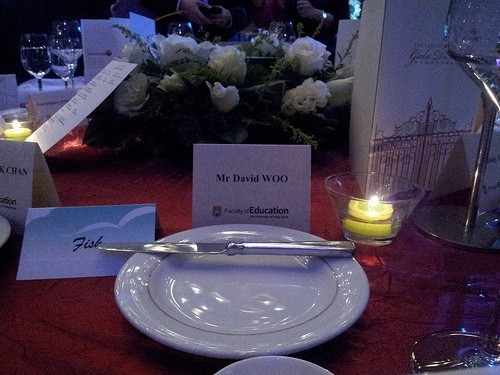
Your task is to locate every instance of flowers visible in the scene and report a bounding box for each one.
[115,32,331,117]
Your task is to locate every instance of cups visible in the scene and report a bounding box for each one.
[166,22,192,39]
[270,21,295,44]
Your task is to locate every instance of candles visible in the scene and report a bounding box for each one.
[4,119,32,141]
[343,196,393,238]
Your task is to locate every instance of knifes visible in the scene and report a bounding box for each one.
[98,241,355,257]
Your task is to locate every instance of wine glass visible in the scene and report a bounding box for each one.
[19,33,53,90]
[410,0,500,254]
[408,295,500,375]
[49,20,83,88]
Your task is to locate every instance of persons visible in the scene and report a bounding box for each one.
[141,0,349,36]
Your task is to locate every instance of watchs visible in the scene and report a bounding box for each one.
[320,9,329,24]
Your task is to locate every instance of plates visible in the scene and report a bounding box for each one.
[113,224,370,358]
[0,215,11,248]
[212,356,334,375]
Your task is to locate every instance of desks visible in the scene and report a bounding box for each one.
[0,126,500,375]
